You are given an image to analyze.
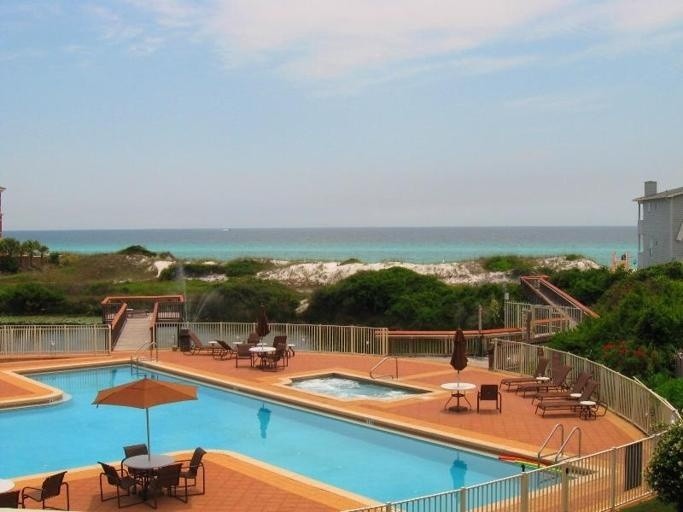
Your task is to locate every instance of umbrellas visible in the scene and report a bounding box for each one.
[449,329,468,412]
[256,304,271,369]
[92,375,199,498]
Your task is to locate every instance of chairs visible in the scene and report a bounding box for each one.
[498,355,609,421]
[0,443,207,512]
[476,384,502,413]
[188,330,295,371]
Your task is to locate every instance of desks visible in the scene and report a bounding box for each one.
[440,382,476,413]
